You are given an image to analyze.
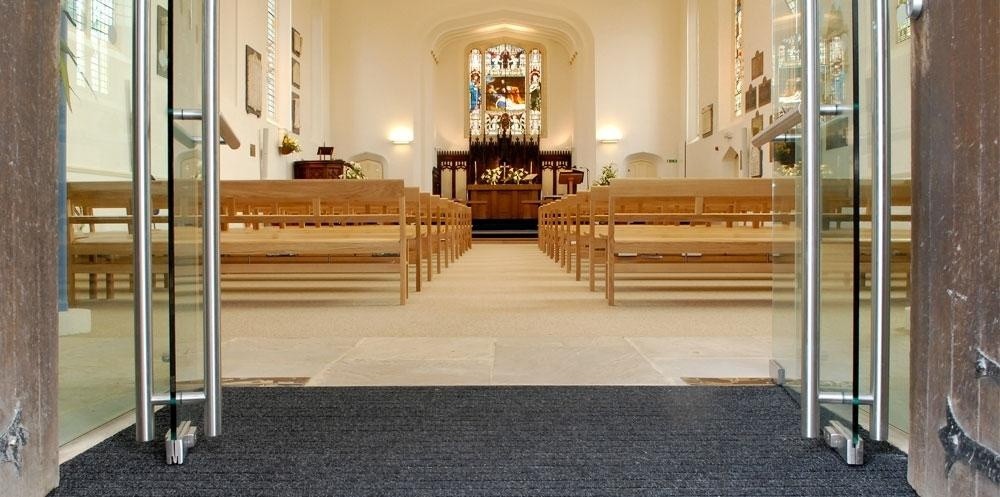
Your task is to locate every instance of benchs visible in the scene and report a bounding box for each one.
[538,177,913,308]
[67,179,473,309]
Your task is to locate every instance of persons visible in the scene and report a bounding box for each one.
[488,78,526,109]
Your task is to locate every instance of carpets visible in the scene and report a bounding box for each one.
[42,386,916,495]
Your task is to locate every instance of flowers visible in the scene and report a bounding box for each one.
[508,168,528,181]
[481,168,502,183]
[340,161,364,179]
[281,137,302,152]
[777,160,802,176]
[597,161,618,185]
